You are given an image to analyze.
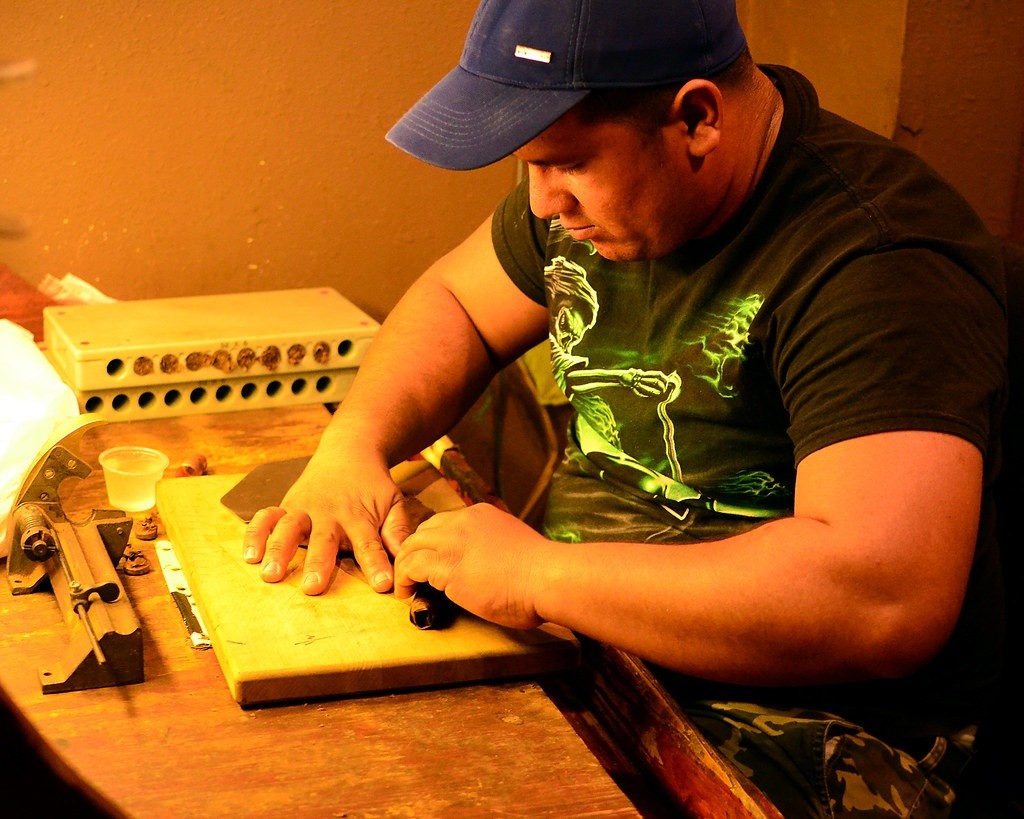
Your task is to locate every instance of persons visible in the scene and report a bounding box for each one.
[243,0,1024,819]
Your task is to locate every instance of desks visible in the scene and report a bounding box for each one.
[0,403,786,819]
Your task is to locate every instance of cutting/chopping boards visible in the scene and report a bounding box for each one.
[149,463,586,709]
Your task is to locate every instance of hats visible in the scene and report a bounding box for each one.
[384,0,747,171]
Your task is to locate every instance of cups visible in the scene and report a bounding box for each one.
[97,447,168,511]
